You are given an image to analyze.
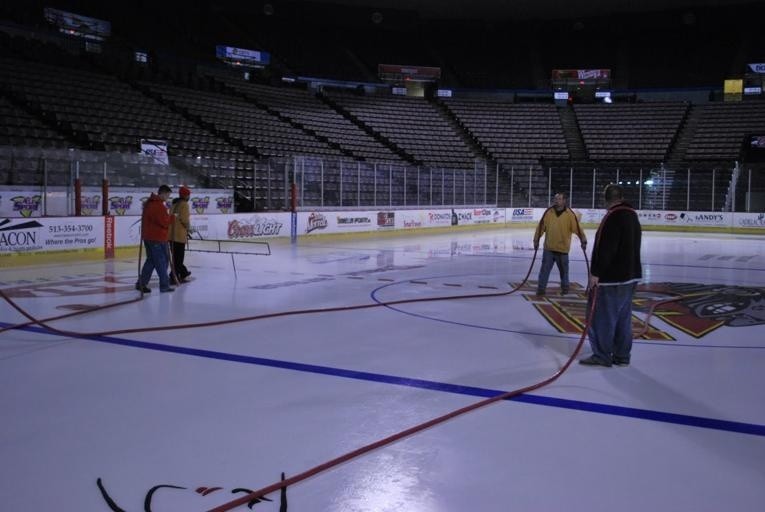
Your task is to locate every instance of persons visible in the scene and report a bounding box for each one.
[615,92,641,104]
[578,184,643,367]
[168,186,191,285]
[533,191,587,295]
[135,185,180,292]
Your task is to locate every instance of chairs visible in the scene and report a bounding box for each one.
[1,54,765,211]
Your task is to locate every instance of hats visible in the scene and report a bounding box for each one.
[179,188,190,198]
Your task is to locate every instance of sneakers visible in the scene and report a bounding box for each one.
[160,288,174,291]
[537,291,545,295]
[170,272,191,282]
[609,355,630,365]
[136,284,151,292]
[561,289,568,294]
[580,357,612,366]
